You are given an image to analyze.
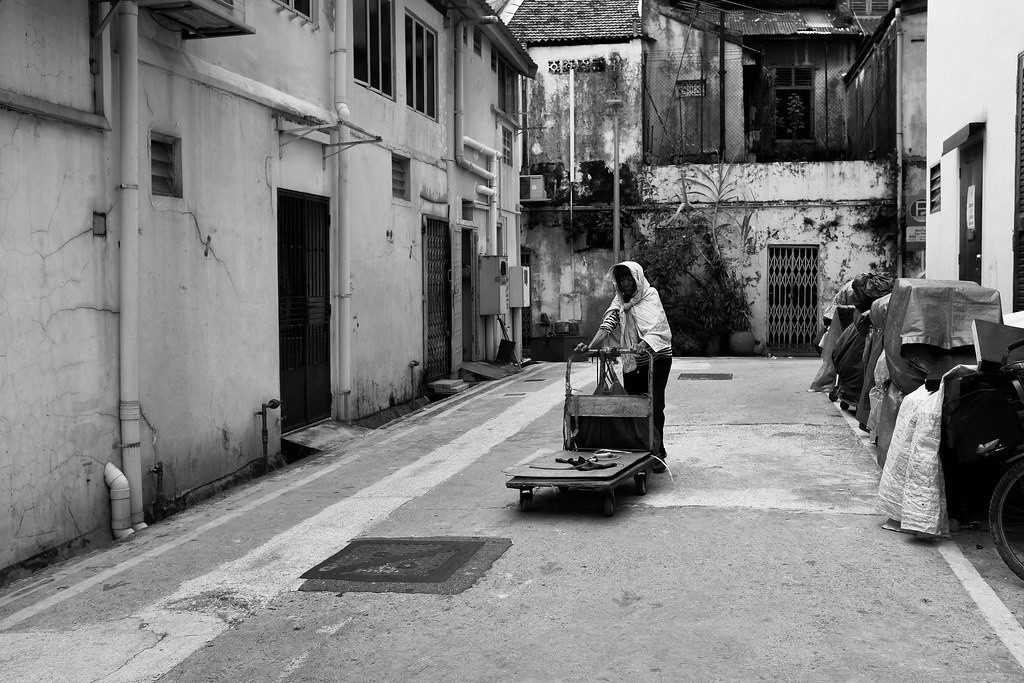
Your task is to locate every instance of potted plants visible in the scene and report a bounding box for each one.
[633,151,755,356]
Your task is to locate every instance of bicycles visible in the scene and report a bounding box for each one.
[939,338,1024,586]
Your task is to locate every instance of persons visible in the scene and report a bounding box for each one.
[575,262,672,473]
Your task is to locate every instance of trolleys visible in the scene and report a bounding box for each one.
[504,348,663,517]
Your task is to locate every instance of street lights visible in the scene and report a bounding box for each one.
[605,97,626,294]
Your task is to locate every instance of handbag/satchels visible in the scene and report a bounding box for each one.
[569,352,667,460]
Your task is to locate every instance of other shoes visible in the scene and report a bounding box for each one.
[650,459,667,473]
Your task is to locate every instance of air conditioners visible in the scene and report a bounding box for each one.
[137,0,245,34]
[518,174,547,201]
